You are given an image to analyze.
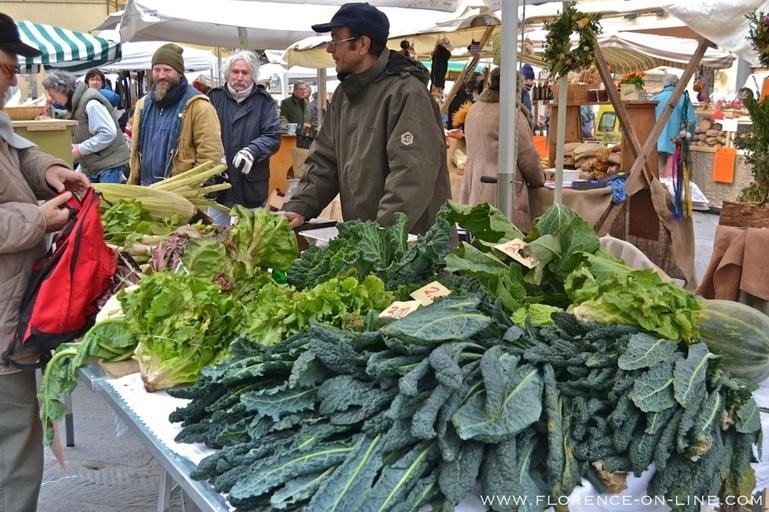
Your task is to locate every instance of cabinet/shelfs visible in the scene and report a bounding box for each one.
[546,100,660,242]
[688,109,748,154]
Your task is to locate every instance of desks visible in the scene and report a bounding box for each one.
[269,134,297,201]
[9,117,79,170]
[527,186,617,238]
[48,343,225,509]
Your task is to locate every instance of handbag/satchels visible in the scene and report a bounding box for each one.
[18,187,118,352]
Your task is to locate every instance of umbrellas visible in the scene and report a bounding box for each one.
[252,0,668,243]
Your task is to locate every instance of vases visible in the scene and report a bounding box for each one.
[620,84,639,100]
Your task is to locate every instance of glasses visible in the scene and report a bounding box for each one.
[327,37,356,49]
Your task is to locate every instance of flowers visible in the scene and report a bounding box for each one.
[619,71,646,90]
[542,1,602,84]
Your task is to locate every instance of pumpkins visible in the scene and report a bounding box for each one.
[696,299,769,386]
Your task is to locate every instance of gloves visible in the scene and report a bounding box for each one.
[232,147,255,175]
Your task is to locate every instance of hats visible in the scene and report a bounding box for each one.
[0,13,42,57]
[312,3,389,37]
[152,43,184,74]
[522,64,534,79]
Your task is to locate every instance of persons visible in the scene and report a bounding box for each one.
[84,68,106,90]
[127,43,225,187]
[278,80,331,130]
[203,49,281,208]
[41,68,131,183]
[650,74,697,179]
[0,10,91,512]
[276,3,460,250]
[446,62,548,240]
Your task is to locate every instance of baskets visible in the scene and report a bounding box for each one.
[550,66,591,104]
